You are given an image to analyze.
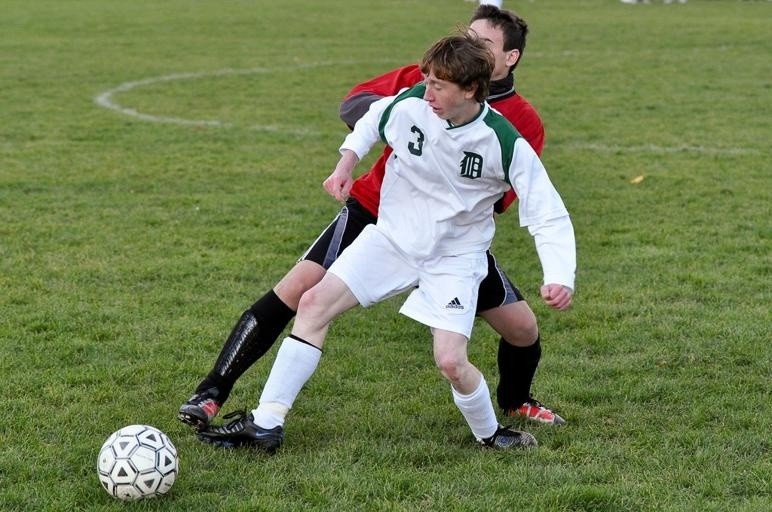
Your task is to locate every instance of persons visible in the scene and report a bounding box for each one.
[193,22,577,457]
[179,2,567,429]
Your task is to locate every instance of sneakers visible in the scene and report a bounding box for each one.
[179,392,219,430]
[472,425,537,452]
[503,401,566,426]
[196,405,284,455]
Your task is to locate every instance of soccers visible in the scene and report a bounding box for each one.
[96,425,179,503]
